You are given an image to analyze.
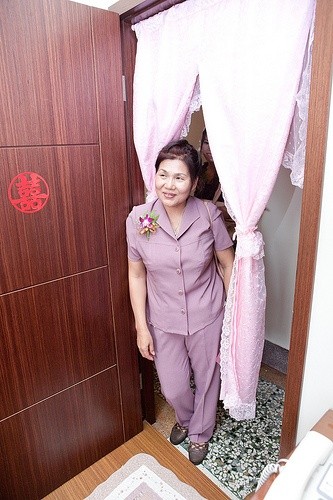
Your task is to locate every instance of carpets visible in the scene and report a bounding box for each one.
[82,453,205,500]
[153,360,285,500]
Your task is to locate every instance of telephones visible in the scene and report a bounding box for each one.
[260,430,332,500]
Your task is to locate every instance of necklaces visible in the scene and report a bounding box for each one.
[174,220,181,236]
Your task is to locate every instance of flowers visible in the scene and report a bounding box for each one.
[137,211,159,237]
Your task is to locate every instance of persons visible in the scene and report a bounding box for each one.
[125,139,235,464]
[198,128,237,251]
